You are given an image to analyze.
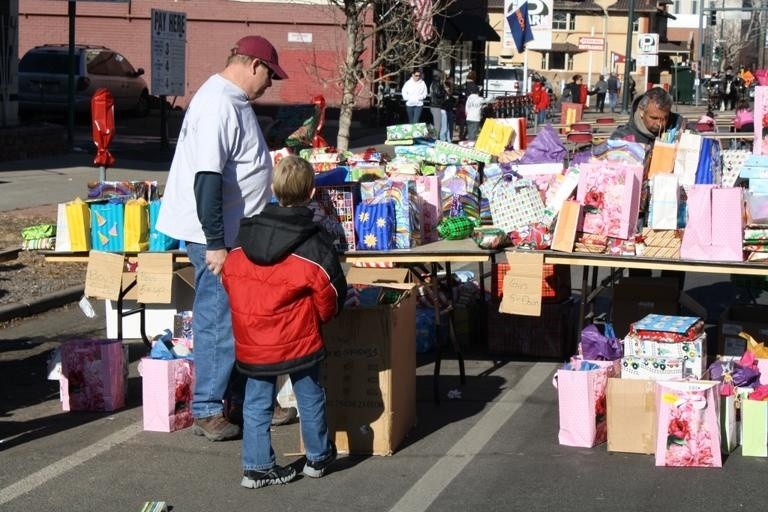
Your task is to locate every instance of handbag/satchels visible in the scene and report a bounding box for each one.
[141,358,194,433]
[553,340,768,467]
[487,162,643,253]
[56,200,180,253]
[58,340,130,412]
[649,119,743,264]
[356,177,441,251]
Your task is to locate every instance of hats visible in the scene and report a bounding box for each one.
[231,35,288,80]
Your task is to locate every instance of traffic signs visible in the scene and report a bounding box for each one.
[579,37,605,46]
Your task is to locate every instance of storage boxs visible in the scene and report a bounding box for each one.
[611,277,681,339]
[297,266,418,459]
[718,303,768,350]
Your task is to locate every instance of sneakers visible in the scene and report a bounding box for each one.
[272,404,297,425]
[192,415,240,441]
[302,440,337,477]
[240,466,296,488]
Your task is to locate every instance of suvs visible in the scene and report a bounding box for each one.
[19,44,149,127]
[483,64,554,105]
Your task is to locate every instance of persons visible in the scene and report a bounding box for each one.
[609,87,687,142]
[401,68,495,143]
[696,65,755,133]
[156,34,299,443]
[223,157,345,488]
[529,72,635,124]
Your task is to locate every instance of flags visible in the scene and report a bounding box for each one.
[505,0,535,55]
[405,1,435,42]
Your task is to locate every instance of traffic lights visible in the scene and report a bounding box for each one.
[710,11,717,26]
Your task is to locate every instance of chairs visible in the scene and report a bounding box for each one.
[564,116,615,154]
[693,121,755,133]
